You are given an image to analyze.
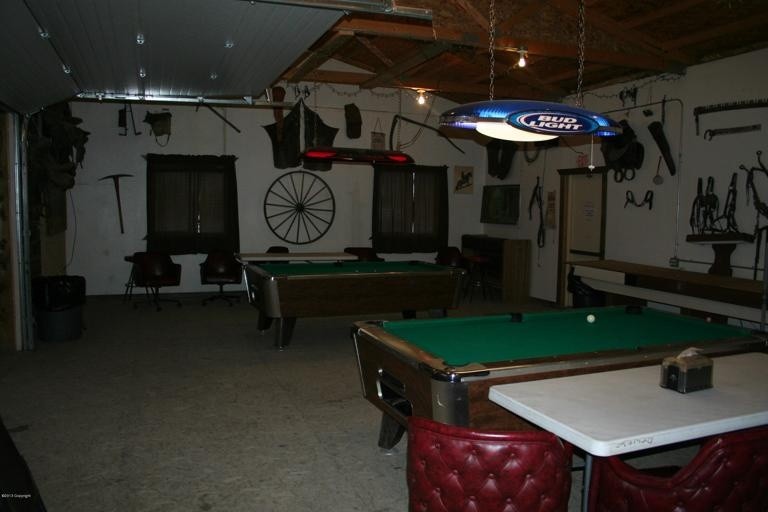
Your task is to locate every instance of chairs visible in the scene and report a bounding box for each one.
[585,425,768,510]
[402,414,572,510]
[248,244,289,264]
[436,235,493,303]
[125,251,183,310]
[200,246,243,307]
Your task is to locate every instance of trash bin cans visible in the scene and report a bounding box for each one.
[33,275,85,342]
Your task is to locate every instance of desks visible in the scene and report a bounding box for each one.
[487,348,766,511]
[246,260,460,354]
[564,259,767,331]
[350,303,766,452]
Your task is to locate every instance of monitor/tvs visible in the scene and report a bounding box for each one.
[480,184,520,226]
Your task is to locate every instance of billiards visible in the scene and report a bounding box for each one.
[705,316,712,323]
[587,314,596,323]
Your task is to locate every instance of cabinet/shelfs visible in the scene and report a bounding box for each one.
[462,233,531,306]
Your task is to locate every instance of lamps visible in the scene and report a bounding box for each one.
[440,1,623,148]
[301,71,416,165]
